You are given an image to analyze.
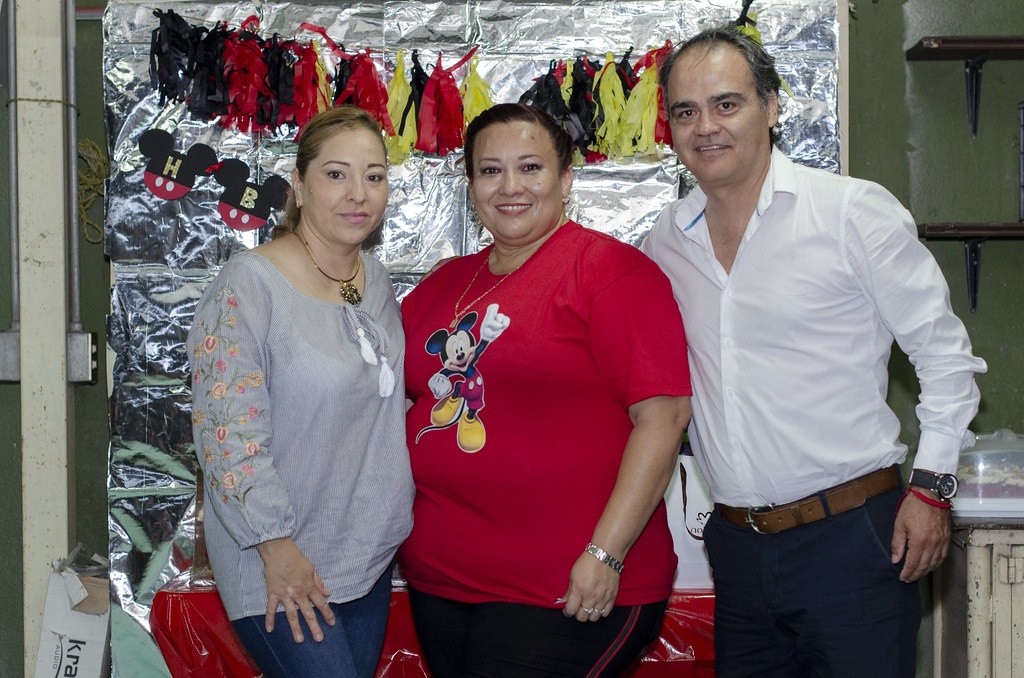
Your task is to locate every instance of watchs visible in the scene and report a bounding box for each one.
[909,468,960,504]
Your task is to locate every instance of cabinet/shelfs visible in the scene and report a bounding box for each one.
[906,33,1023,312]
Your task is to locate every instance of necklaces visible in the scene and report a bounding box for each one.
[450,248,537,328]
[292,231,364,306]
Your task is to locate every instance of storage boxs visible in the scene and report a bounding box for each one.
[34,556,114,678]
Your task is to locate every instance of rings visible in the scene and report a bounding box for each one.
[593,607,605,613]
[580,605,594,614]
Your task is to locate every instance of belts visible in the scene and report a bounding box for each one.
[717,463,899,535]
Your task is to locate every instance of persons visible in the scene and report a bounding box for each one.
[645,27,987,678]
[393,102,693,678]
[187,108,417,678]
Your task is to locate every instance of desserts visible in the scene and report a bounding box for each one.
[953,461,1024,498]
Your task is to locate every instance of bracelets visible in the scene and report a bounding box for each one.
[895,488,952,519]
[583,542,624,574]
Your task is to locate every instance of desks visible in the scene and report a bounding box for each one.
[147,571,714,678]
